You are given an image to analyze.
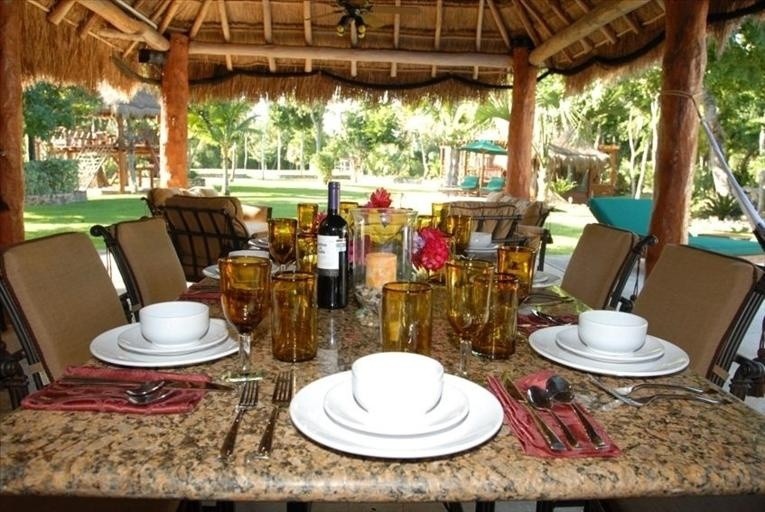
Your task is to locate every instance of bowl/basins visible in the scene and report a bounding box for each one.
[352,351,444,415]
[470,231,491,245]
[579,309,648,352]
[138,301,209,345]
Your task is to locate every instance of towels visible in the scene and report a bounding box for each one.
[182,271,224,303]
[483,368,619,457]
[20,364,208,416]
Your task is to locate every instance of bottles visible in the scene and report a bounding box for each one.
[316,181,349,308]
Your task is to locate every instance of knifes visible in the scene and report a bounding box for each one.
[57,378,234,395]
[506,375,563,451]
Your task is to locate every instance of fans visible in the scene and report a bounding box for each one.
[304,0,421,29]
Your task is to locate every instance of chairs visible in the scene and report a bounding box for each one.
[460,175,478,197]
[1,230,127,410]
[164,193,271,282]
[187,186,272,225]
[142,188,179,214]
[589,197,765,257]
[90,216,189,319]
[559,222,655,311]
[630,244,764,402]
[481,176,504,196]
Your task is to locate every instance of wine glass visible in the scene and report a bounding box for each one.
[297,201,317,235]
[445,260,496,378]
[450,215,472,259]
[267,218,298,275]
[217,255,274,383]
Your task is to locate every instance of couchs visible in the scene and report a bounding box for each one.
[440,201,540,258]
[490,193,549,271]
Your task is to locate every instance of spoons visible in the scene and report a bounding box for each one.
[43,389,178,408]
[547,314,579,324]
[529,386,580,450]
[546,377,606,448]
[53,380,166,399]
[530,307,581,318]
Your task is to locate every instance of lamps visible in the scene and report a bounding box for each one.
[336,8,367,39]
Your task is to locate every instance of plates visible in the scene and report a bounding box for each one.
[289,371,504,459]
[528,324,690,378]
[532,270,550,283]
[464,245,505,250]
[323,380,468,436]
[535,270,563,291]
[203,263,228,281]
[555,326,666,362]
[89,316,245,366]
[117,321,230,355]
[462,249,503,258]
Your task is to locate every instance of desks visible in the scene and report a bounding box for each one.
[0,231,764,511]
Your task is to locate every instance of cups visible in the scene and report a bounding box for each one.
[295,234,317,275]
[273,270,319,363]
[335,204,443,251]
[381,280,433,358]
[472,276,518,358]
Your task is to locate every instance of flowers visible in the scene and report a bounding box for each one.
[312,188,448,282]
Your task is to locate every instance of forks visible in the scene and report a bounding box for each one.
[221,380,276,449]
[587,373,719,395]
[258,371,296,453]
[599,381,727,408]
